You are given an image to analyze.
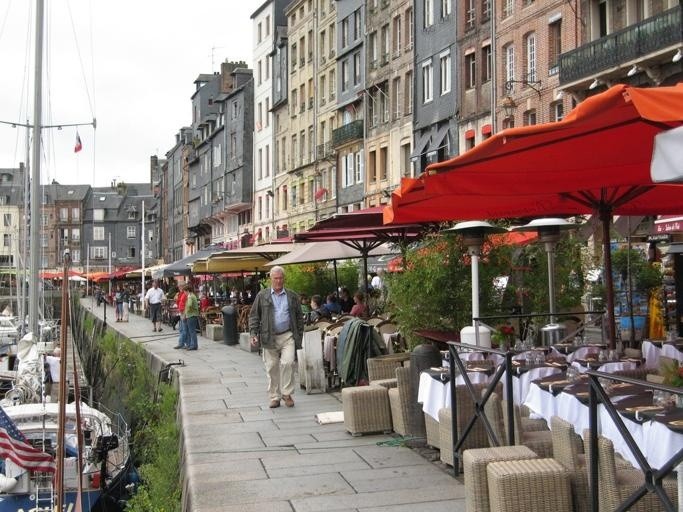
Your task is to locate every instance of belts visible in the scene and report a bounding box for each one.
[276,328,291,335]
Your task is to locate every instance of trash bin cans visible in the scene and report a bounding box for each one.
[408,344,442,437]
[221,306,238,345]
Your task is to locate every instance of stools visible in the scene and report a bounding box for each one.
[486,458,574,511]
[462,445,540,511]
[339,384,393,439]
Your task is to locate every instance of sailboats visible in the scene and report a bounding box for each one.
[1,0,143,512]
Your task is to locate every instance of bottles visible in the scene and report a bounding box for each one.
[565,366,579,383]
[524,352,545,370]
[653,390,671,413]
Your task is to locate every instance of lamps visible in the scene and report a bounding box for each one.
[499,78,543,120]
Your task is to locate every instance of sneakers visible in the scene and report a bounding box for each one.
[269,400,280,408]
[282,394,294,408]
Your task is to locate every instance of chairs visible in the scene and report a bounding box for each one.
[437,383,503,471]
[501,400,552,458]
[481,388,551,446]
[366,354,402,392]
[643,419,682,473]
[554,393,590,441]
[387,367,412,439]
[440,340,683,418]
[418,372,444,449]
[596,403,645,471]
[552,415,589,511]
[128,294,398,395]
[584,428,679,510]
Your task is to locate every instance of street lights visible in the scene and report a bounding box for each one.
[439,223,510,354]
[510,219,582,350]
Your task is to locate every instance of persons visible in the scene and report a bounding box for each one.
[292,271,392,324]
[67,279,259,350]
[248,265,303,409]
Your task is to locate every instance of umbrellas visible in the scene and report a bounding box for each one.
[0,241,295,283]
[381,81,683,352]
[269,238,407,303]
[292,199,474,303]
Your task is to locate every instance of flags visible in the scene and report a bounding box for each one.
[73,129,84,152]
[0,404,55,484]
